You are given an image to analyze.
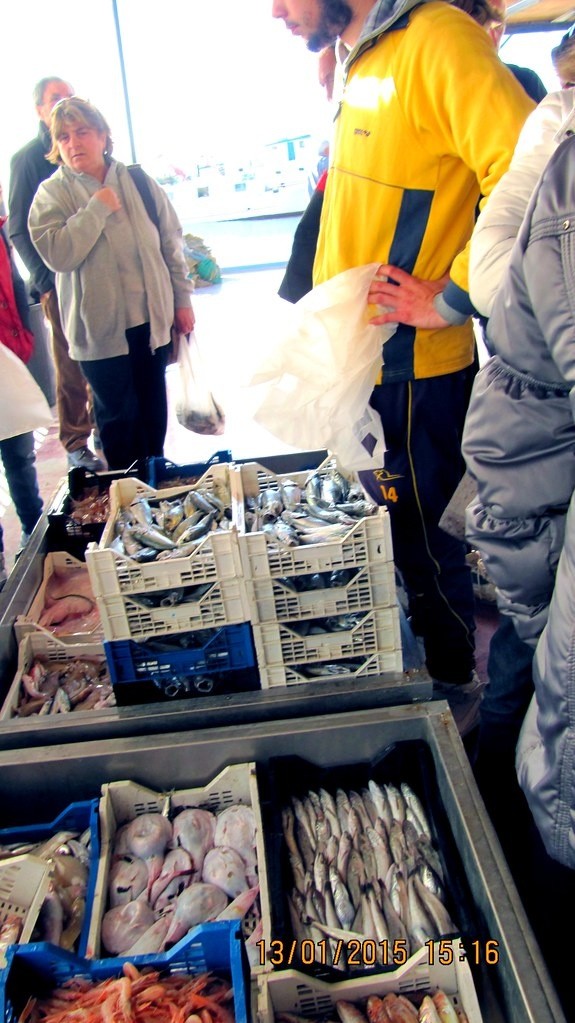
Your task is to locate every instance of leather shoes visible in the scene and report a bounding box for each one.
[95,440,104,450]
[68,446,103,470]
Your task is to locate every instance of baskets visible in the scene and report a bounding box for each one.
[0,762,481,1023]
[1,450,406,727]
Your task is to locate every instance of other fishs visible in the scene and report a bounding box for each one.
[10,470,378,720]
[101,803,263,964]
[276,775,467,1021]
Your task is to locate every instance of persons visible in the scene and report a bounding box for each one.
[0,186,44,594]
[272,0,575,1023]
[8,76,112,469]
[28,96,194,471]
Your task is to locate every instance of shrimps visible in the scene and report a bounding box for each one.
[16,960,234,1022]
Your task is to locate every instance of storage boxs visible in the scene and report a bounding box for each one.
[0,736,487,1023]
[0,452,405,722]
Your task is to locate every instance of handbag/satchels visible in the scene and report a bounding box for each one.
[175,334,226,436]
[128,163,191,362]
